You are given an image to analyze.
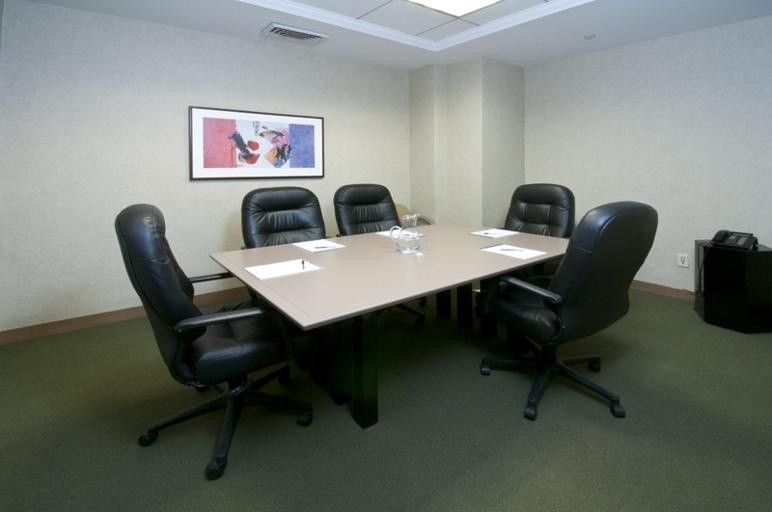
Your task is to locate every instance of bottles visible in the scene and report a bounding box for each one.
[389,213,426,256]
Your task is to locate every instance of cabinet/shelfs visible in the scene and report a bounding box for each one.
[695,239,772,334]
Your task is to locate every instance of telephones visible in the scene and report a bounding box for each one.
[710,229,757,251]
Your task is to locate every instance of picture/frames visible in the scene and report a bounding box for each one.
[188,106,325,180]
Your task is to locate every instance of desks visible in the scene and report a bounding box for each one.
[209,222,571,429]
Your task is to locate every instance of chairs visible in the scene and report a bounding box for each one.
[480,199,656,421]
[116,201,314,480]
[242,186,328,300]
[333,183,427,320]
[497,183,576,277]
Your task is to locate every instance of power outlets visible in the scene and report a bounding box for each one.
[677,253,689,268]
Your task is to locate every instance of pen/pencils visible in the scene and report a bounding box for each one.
[301,259,305,269]
[501,248,522,251]
[484,232,497,234]
[315,247,328,248]
